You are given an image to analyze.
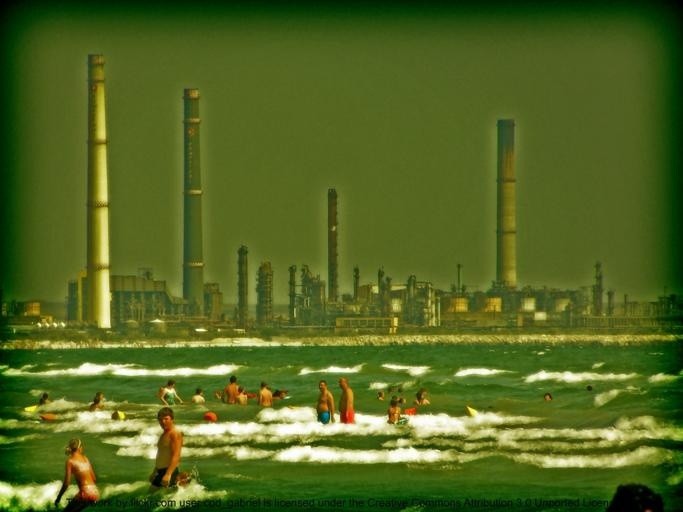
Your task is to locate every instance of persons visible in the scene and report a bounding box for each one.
[544,393,552,400]
[148,407,182,495]
[39,375,287,412]
[378,385,430,424]
[338,377,355,424]
[50,439,100,511]
[317,381,335,424]
[606,483,664,512]
